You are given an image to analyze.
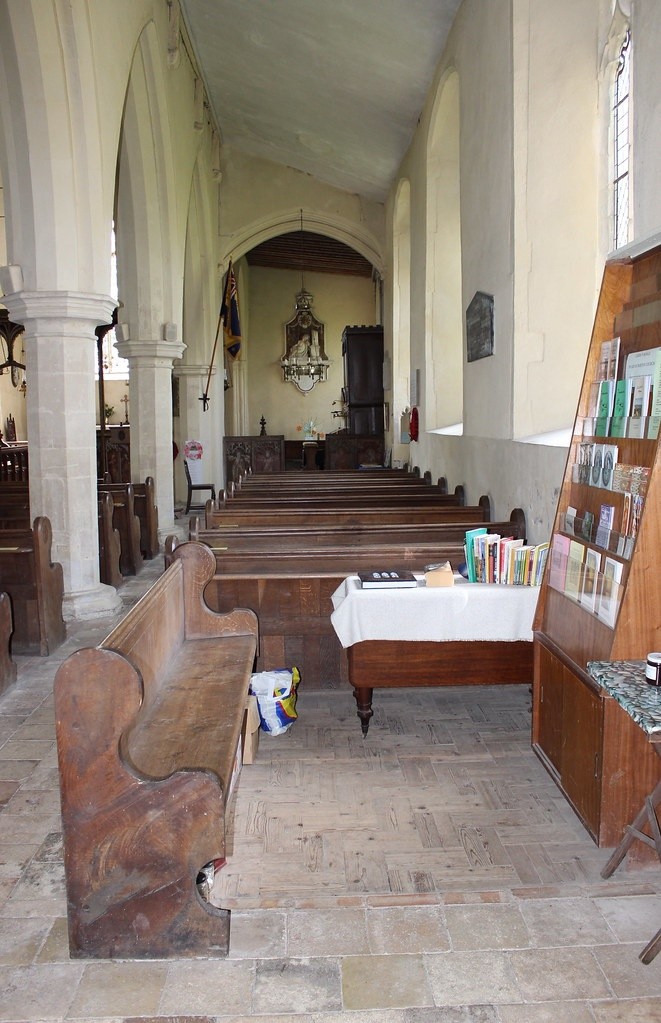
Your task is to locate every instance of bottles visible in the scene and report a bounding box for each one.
[196,872,209,903]
[199,861,214,889]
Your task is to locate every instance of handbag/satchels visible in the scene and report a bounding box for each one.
[249,667,300,736]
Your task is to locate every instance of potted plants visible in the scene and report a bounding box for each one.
[105,403,115,422]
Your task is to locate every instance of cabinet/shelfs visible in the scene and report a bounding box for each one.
[532,251,661,849]
[226,435,284,471]
[323,324,384,470]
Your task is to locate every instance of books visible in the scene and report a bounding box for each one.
[547,533,623,628]
[581,337,651,439]
[462,527,550,586]
[358,570,417,588]
[572,442,619,490]
[565,504,615,550]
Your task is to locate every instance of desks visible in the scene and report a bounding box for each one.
[587,661,661,965]
[328,574,541,739]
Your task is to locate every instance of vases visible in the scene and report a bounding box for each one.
[304,432,314,440]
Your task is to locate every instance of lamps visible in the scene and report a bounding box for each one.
[294,209,314,311]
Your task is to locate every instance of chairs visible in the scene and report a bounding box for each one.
[183,460,215,514]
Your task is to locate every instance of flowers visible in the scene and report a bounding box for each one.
[296,416,324,438]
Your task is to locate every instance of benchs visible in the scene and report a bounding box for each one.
[0,464,526,958]
